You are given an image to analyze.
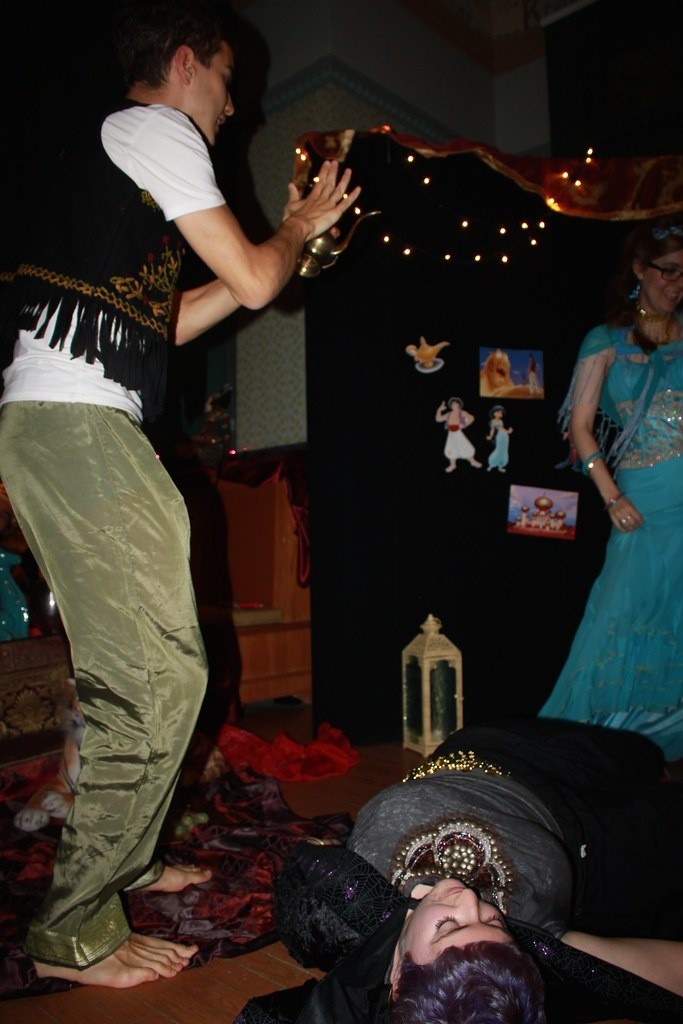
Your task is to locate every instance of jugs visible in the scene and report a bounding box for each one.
[290,210,381,294]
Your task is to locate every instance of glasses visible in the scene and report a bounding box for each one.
[642,256,683,282]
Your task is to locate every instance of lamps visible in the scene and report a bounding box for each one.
[400,612,464,760]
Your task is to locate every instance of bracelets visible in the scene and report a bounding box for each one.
[304,836,324,846]
[604,492,625,512]
[582,452,605,476]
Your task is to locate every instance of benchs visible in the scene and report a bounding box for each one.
[0,463,313,738]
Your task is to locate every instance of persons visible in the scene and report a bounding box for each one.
[270,715,683,1024]
[539,218,683,773]
[0,0,360,990]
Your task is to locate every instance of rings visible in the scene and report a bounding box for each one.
[621,519,626,524]
[627,515,631,519]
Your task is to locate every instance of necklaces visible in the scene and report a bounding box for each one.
[388,815,512,917]
